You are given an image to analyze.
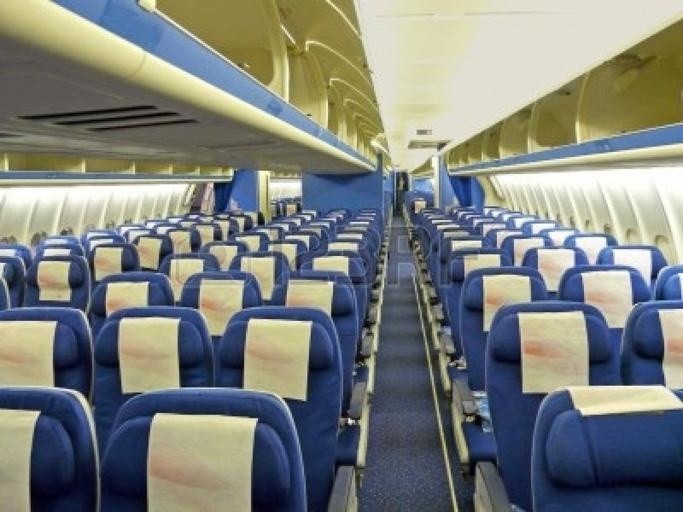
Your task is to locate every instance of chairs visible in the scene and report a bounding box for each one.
[531,384,683,512]
[267,268,359,427]
[439,249,514,400]
[596,245,667,299]
[565,233,616,267]
[654,267,683,302]
[473,302,620,512]
[408,200,581,351]
[450,267,549,478]
[214,306,360,512]
[522,245,590,300]
[556,264,651,358]
[99,386,312,511]
[622,301,683,391]
[94,306,215,458]
[0,385,97,512]
